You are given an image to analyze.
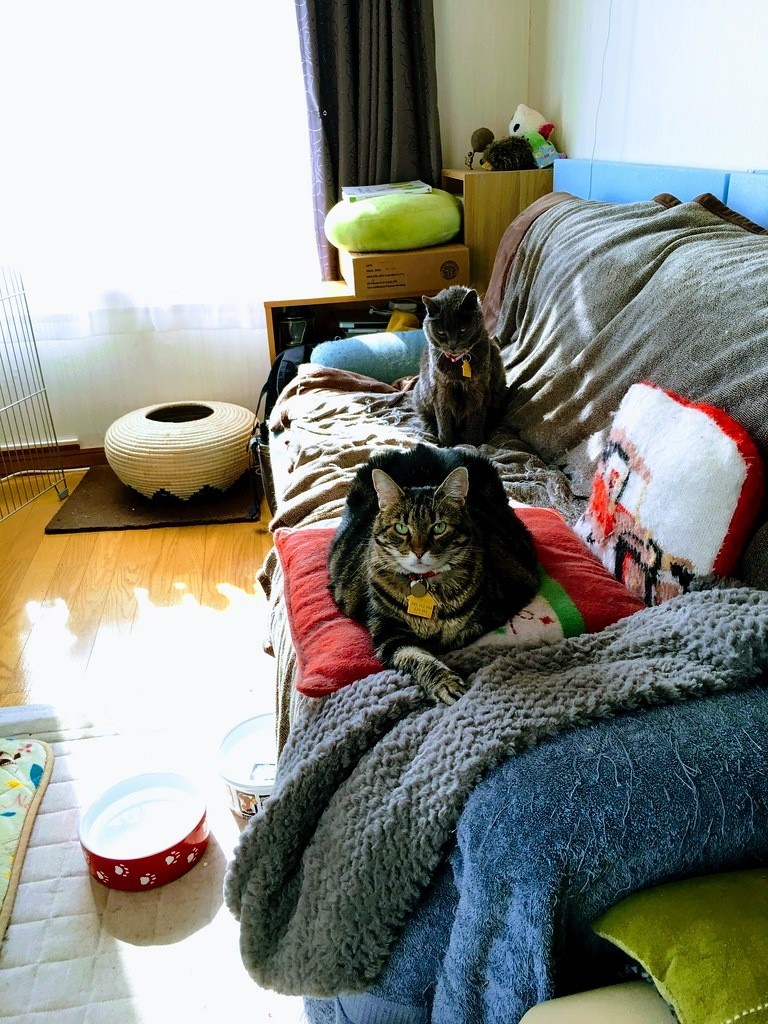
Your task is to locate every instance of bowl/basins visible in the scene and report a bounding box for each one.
[215,712,277,821]
[77,771,212,892]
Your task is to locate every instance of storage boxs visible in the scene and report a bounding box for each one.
[335,240,470,296]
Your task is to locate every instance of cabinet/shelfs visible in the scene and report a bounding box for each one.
[261,162,555,364]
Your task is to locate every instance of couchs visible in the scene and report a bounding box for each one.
[270,188,768,1023]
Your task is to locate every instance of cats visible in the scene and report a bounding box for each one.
[325,444,545,712]
[410,284,508,448]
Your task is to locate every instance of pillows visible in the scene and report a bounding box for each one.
[322,186,464,254]
[272,504,659,699]
[574,376,761,609]
[309,327,427,385]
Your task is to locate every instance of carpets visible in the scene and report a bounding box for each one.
[1,705,306,1023]
[44,463,265,538]
